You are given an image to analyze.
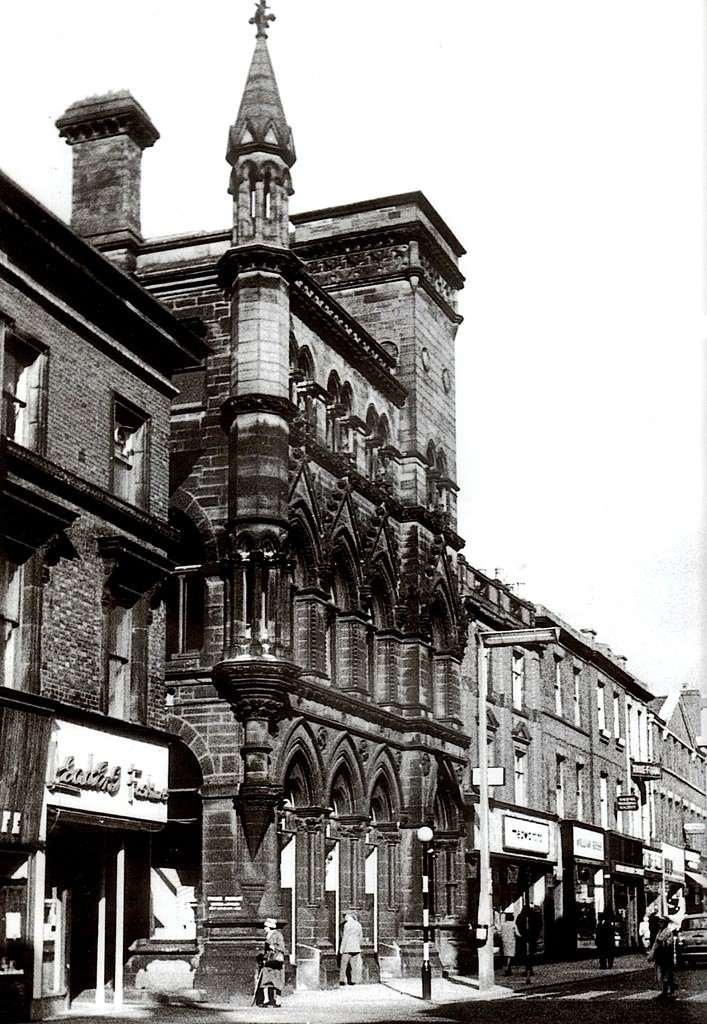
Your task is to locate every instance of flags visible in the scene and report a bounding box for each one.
[365,851,377,894]
[325,846,339,891]
[281,840,296,889]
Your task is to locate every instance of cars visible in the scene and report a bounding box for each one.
[672,912,707,969]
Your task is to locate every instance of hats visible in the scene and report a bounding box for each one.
[264,918,277,928]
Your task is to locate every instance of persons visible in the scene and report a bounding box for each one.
[595,912,617,970]
[501,913,522,976]
[339,910,363,985]
[639,915,650,949]
[647,915,674,1004]
[258,917,286,1008]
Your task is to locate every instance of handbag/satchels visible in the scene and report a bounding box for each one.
[267,950,284,961]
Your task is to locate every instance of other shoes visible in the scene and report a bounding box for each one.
[275,1001,281,1007]
[259,1001,270,1007]
[504,970,513,976]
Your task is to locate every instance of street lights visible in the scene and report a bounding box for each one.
[417,826,435,1001]
